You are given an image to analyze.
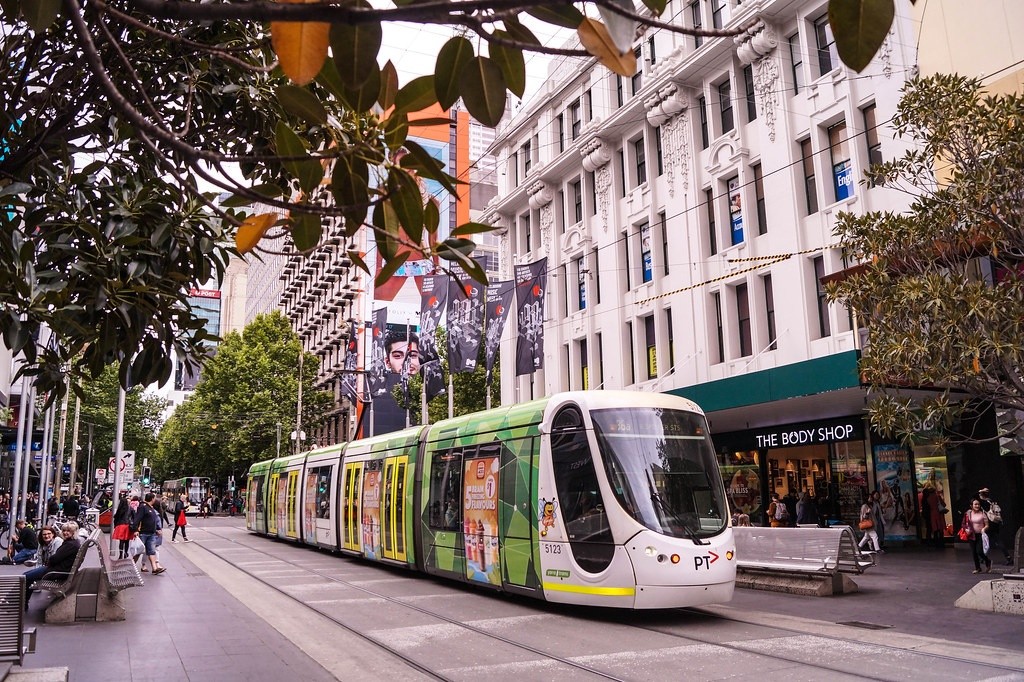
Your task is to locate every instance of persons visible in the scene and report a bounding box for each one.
[172,495,193,543]
[857,490,887,554]
[385,332,420,374]
[961,487,1014,574]
[766,488,813,527]
[0,488,174,612]
[731,509,750,527]
[196,491,243,518]
[922,487,947,549]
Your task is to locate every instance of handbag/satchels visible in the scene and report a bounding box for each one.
[981,532,990,556]
[957,515,970,542]
[128,536,147,557]
[936,494,948,514]
[859,508,874,530]
[177,510,187,526]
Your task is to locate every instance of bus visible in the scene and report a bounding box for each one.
[161,475,213,515]
[246,389,740,612]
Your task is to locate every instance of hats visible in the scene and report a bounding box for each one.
[162,496,168,499]
[978,487,989,493]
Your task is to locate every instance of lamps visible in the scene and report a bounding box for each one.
[811,463,819,472]
[785,459,795,472]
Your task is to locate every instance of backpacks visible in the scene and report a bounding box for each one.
[771,501,790,521]
[981,500,1003,525]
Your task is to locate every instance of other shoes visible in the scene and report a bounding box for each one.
[118,555,122,560]
[168,523,174,527]
[124,552,129,559]
[141,567,150,573]
[972,567,982,573]
[986,559,993,573]
[183,537,193,543]
[152,568,167,575]
[172,539,179,543]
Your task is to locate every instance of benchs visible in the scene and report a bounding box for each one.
[0,529,145,667]
[734,524,877,595]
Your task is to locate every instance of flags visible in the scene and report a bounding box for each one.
[419,256,548,404]
[340,306,388,399]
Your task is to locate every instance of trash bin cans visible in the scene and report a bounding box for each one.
[86,506,100,528]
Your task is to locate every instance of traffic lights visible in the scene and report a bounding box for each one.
[142,466,152,487]
[126,483,132,490]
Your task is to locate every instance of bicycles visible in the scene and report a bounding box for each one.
[0,505,99,551]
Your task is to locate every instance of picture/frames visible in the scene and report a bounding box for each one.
[770,458,815,498]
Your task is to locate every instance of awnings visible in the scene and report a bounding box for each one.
[10,463,40,478]
